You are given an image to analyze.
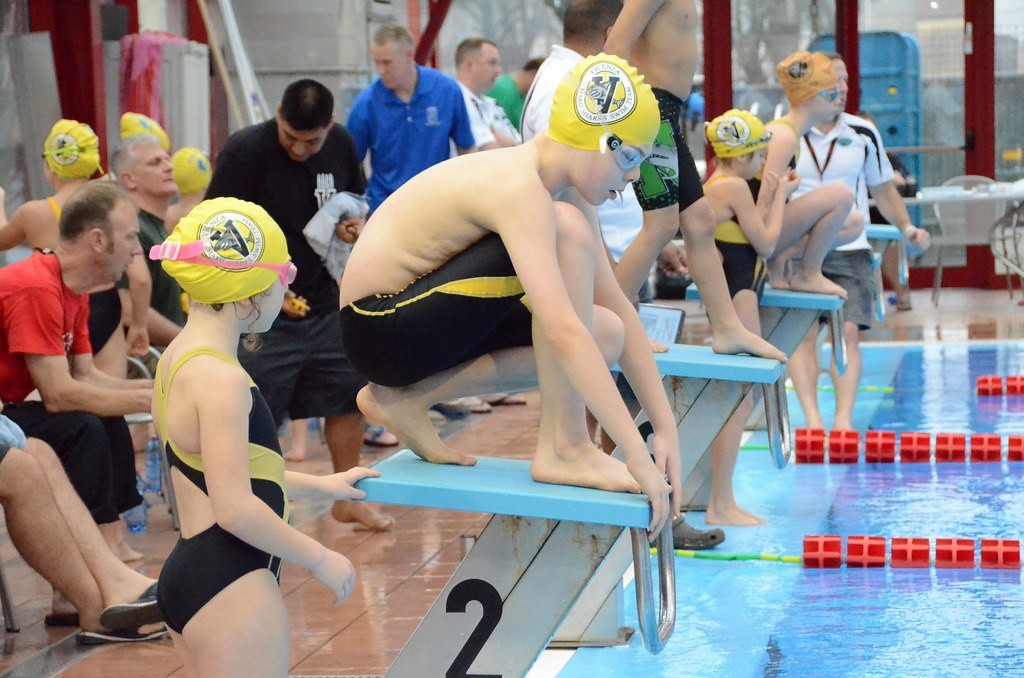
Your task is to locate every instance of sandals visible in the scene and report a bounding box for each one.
[649,517,725,550]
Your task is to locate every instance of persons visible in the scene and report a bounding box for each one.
[0,400,169,648]
[867,151,918,311]
[339,53,682,543]
[597,0,791,368]
[0,105,216,378]
[1,178,153,623]
[206,29,547,532]
[700,110,800,530]
[786,52,932,432]
[147,198,381,678]
[749,50,866,299]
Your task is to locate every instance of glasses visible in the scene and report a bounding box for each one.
[760,132,772,141]
[274,263,298,285]
[606,131,643,169]
[819,90,839,101]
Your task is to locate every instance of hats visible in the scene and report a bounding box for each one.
[706,107,769,157]
[120,113,170,151]
[544,51,660,151]
[162,197,291,304]
[172,147,213,193]
[777,52,836,103]
[44,119,100,177]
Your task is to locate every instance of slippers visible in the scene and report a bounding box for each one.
[467,400,491,414]
[492,394,527,406]
[45,613,79,628]
[76,619,168,646]
[99,582,160,628]
[364,426,399,447]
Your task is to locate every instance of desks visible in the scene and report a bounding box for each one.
[864,192,1024,308]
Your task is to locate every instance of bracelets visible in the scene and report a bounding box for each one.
[899,224,916,236]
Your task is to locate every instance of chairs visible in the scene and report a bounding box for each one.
[931,174,1015,306]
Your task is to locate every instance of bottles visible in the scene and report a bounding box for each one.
[307,418,320,443]
[123,501,147,533]
[146,437,161,492]
[136,473,147,495]
